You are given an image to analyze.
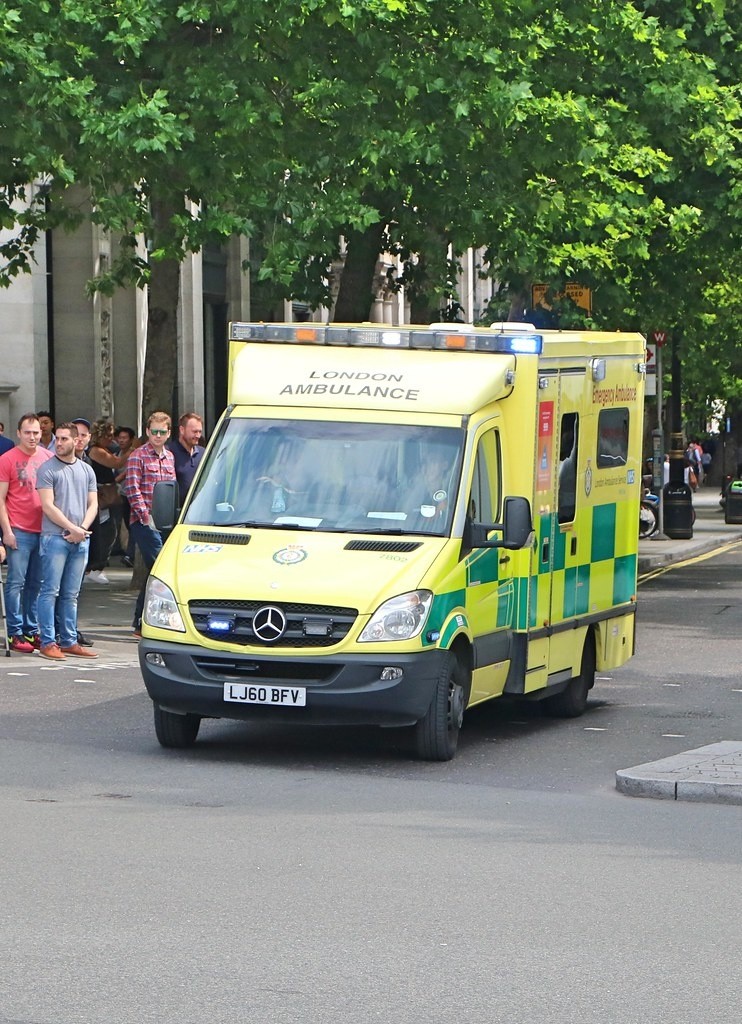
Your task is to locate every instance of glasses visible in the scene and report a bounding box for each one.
[148,429,168,435]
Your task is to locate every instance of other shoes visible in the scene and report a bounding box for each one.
[121,556,134,568]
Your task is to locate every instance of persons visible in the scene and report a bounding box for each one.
[645,440,705,496]
[34,422,99,660]
[0,409,208,652]
[405,446,454,530]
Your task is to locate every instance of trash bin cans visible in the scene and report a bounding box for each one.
[663,480,692,540]
[724,479,742,524]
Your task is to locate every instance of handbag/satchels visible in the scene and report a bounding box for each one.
[97,484,125,509]
[689,467,697,488]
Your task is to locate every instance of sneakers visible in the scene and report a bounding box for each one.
[5,635,34,653]
[77,631,94,647]
[24,635,40,648]
[40,643,67,661]
[62,644,100,658]
[89,570,110,584]
[84,575,97,584]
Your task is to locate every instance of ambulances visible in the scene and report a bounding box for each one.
[138,322,648,761]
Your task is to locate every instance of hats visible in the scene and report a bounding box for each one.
[71,417,90,428]
